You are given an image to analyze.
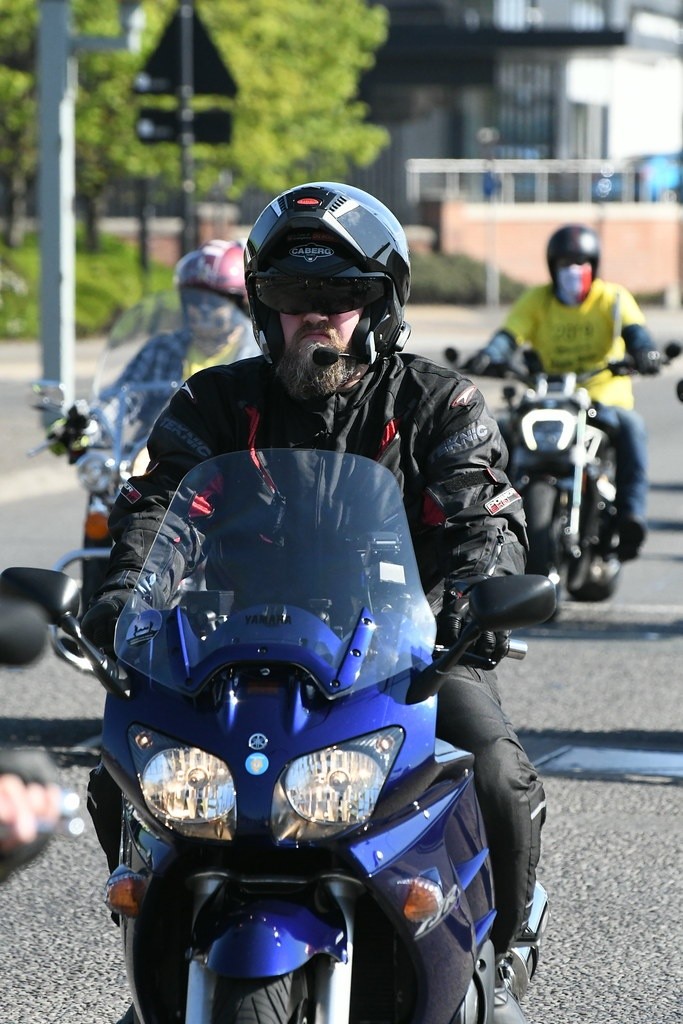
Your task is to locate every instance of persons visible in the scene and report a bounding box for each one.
[466,226,661,560]
[77,181,546,1024]
[47,238,261,660]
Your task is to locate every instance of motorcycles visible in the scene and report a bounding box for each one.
[27,285,265,639]
[443,338,683,603]
[3,447,559,1023]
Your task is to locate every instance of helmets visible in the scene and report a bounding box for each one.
[546,227,599,282]
[176,239,253,316]
[242,181,413,362]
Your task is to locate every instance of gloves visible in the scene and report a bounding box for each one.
[433,573,507,670]
[636,347,662,375]
[469,354,496,376]
[77,588,155,661]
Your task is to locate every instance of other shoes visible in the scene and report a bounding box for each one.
[493,984,526,1024]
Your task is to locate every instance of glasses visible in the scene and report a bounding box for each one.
[257,278,387,315]
[183,292,230,309]
